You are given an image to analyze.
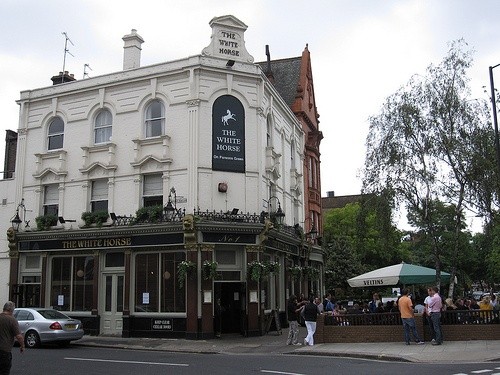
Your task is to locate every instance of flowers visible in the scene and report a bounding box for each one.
[203,259,219,281]
[176,261,196,289]
[288,265,320,282]
[247,260,281,282]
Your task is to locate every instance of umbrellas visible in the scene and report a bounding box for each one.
[347,261,453,312]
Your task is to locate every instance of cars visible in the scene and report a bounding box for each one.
[12,306,85,349]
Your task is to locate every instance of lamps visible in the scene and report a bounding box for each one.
[164,200,175,222]
[11,213,22,232]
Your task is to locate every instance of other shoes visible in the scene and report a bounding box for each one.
[286,342,303,346]
[406,342,410,344]
[304,338,314,346]
[431,339,443,345]
[415,341,425,345]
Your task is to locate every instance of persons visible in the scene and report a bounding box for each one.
[287,287,497,347]
[0,301,25,375]
